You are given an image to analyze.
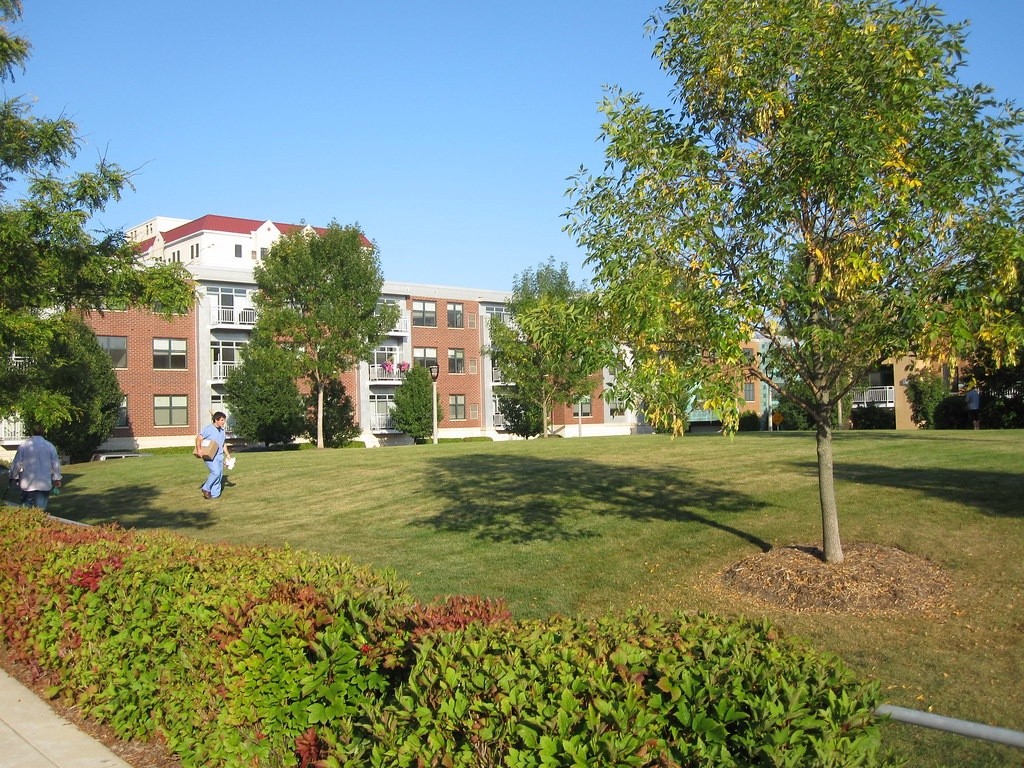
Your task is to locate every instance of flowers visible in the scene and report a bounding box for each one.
[397,361,410,371]
[381,361,392,373]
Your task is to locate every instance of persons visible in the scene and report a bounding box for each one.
[196,411,232,500]
[11,424,62,513]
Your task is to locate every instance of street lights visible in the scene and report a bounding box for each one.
[766,361,778,432]
[429,361,440,444]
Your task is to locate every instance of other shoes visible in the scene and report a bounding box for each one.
[202,488,212,499]
